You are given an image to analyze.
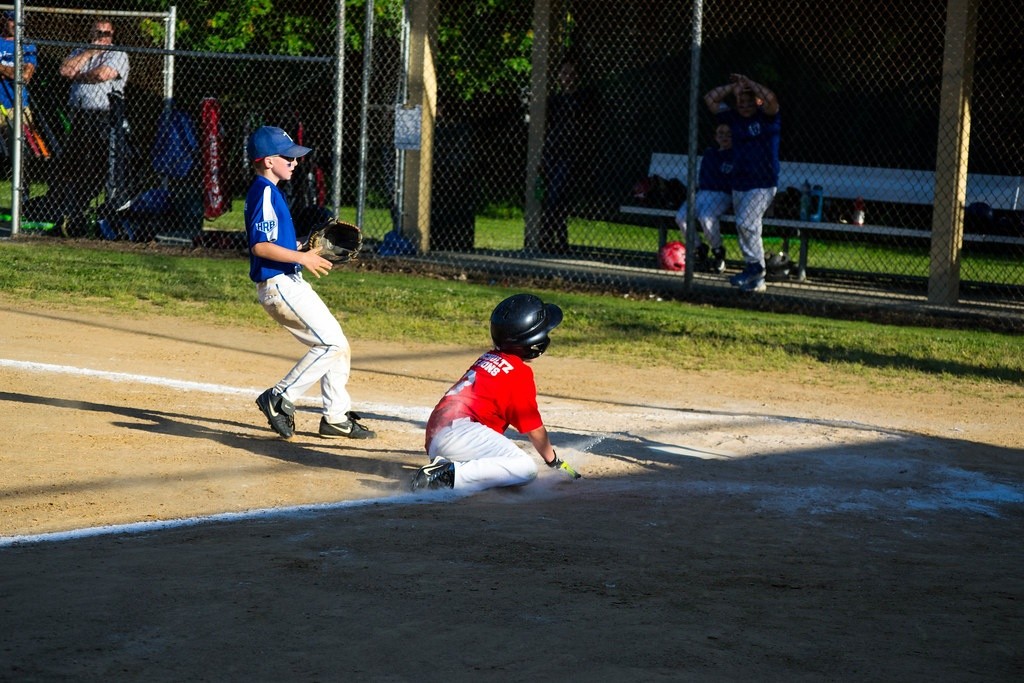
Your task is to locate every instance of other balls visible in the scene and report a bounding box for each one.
[658,240,687,273]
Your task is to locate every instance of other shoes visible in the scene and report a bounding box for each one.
[732,262,767,292]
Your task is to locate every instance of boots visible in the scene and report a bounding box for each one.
[697,243,710,272]
[712,246,725,273]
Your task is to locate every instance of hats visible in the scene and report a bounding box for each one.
[248,126,312,161]
[7,10,15,20]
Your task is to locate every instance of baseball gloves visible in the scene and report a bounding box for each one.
[308,222,363,267]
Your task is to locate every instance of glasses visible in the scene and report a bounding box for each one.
[95,30,111,37]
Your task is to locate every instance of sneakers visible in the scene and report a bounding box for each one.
[410,456,455,491]
[255,387,296,439]
[319,410,377,439]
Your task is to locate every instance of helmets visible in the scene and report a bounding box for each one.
[659,241,685,271]
[491,294,563,359]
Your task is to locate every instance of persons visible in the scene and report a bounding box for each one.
[409,294,581,495]
[674,72,779,290]
[59,21,130,206]
[244,125,377,439]
[0,11,38,182]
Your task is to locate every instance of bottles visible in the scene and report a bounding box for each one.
[854,196,866,223]
[800,180,824,221]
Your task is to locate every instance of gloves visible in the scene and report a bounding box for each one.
[545,449,581,480]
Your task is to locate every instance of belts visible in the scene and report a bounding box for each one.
[284,264,302,275]
[447,418,474,426]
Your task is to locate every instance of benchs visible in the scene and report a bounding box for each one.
[618,153,1023,282]
